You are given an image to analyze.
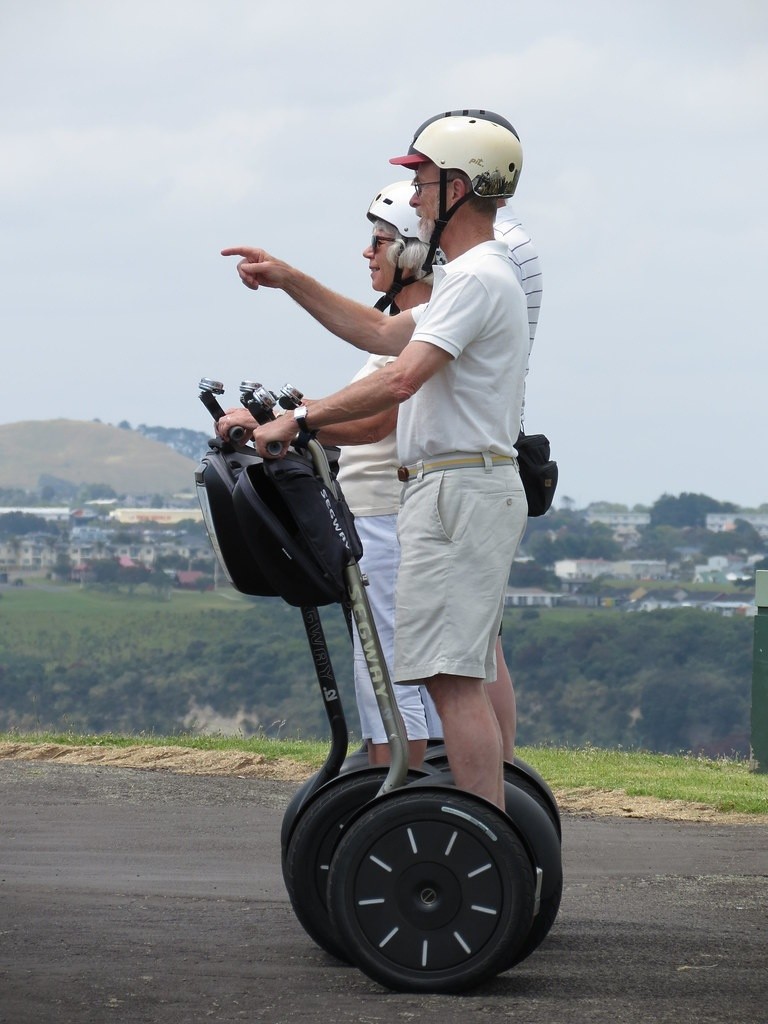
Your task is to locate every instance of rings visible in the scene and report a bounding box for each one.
[227,417,229,419]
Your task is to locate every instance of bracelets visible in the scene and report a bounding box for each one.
[294,404,310,433]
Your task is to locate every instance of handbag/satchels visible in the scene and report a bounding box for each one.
[229,449,368,610]
[517,432,563,519]
[193,437,284,597]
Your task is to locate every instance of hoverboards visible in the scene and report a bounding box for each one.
[194,377,563,992]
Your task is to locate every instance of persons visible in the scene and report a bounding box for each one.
[218,107,544,823]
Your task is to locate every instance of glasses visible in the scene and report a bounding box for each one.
[409,178,453,196]
[371,235,400,250]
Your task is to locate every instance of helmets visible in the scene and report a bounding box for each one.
[388,108,523,204]
[366,179,438,245]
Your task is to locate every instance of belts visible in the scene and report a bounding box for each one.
[394,452,516,483]
